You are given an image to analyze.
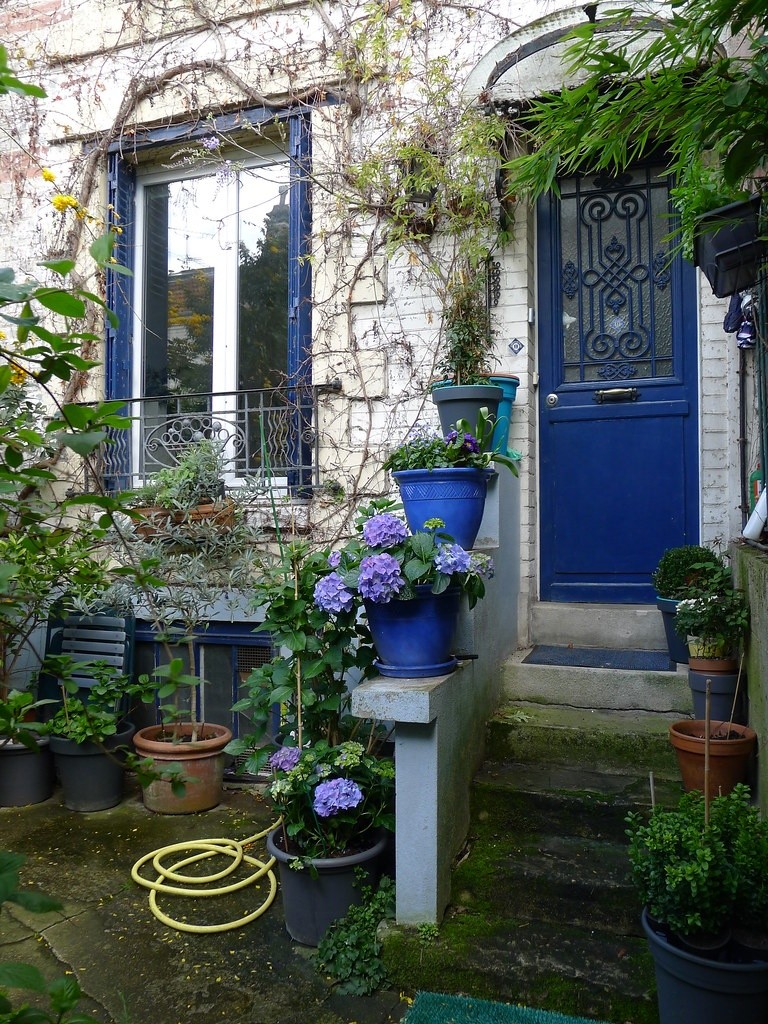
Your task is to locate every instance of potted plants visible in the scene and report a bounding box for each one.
[0,259,286,815]
[670,158,764,298]
[624,781,768,1024]
[652,543,737,657]
[333,0,521,456]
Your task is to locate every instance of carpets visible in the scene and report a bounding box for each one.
[520,644,679,671]
[401,989,613,1023]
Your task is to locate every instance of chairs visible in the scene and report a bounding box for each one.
[36,597,137,718]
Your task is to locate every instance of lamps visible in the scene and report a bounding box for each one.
[400,158,436,209]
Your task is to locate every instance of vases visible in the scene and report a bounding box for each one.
[361,584,462,677]
[670,657,759,802]
[266,822,388,945]
[390,469,490,552]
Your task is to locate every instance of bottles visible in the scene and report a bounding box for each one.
[743,488,768,541]
[748,461,764,517]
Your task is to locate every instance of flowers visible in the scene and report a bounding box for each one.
[672,592,747,661]
[382,405,521,483]
[266,742,397,880]
[312,512,497,613]
